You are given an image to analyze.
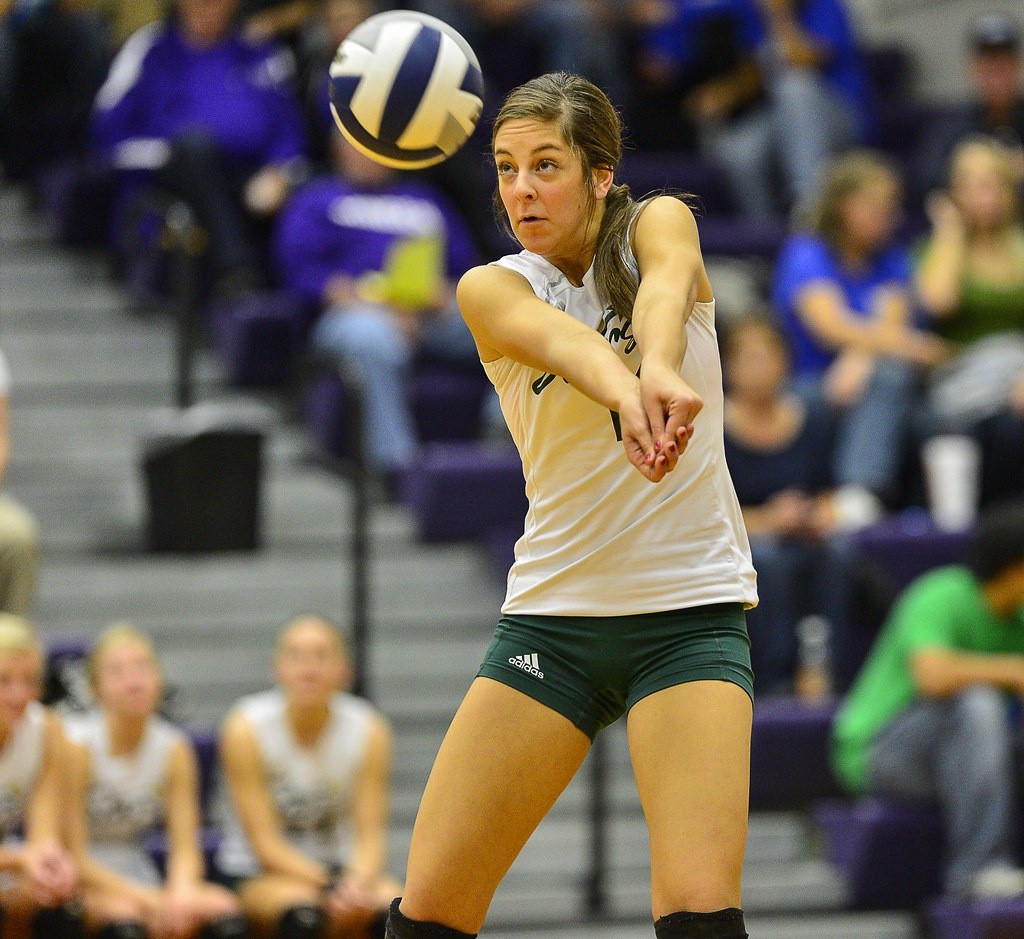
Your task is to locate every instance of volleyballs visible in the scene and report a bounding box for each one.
[322,9,492,172]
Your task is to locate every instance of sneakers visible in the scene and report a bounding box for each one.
[946,863,1024,903]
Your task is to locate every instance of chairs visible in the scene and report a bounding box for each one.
[58,149,952,898]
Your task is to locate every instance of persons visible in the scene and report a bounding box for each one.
[385,71,759,939]
[60,624,245,939]
[0,1,1024,939]
[0,614,76,939]
[213,611,404,938]
